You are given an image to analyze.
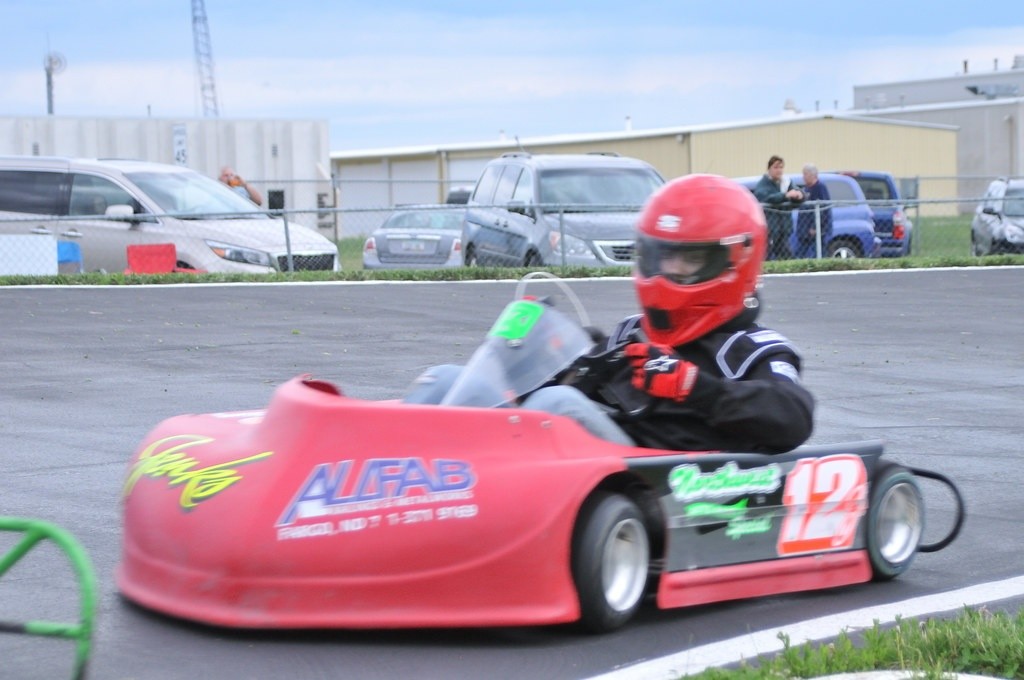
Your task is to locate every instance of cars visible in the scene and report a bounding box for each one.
[362,203,468,270]
[733,173,882,258]
[836,171,913,257]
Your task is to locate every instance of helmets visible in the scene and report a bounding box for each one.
[636,174,768,346]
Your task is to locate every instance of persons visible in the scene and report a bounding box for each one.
[81,193,106,217]
[797,164,833,259]
[217,168,262,205]
[558,172,814,455]
[754,156,810,261]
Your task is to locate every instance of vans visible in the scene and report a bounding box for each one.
[0,155,339,275]
[447,187,472,202]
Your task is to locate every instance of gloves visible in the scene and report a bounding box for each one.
[623,342,699,402]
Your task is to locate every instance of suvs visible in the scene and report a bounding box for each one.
[461,151,667,269]
[971,177,1024,257]
